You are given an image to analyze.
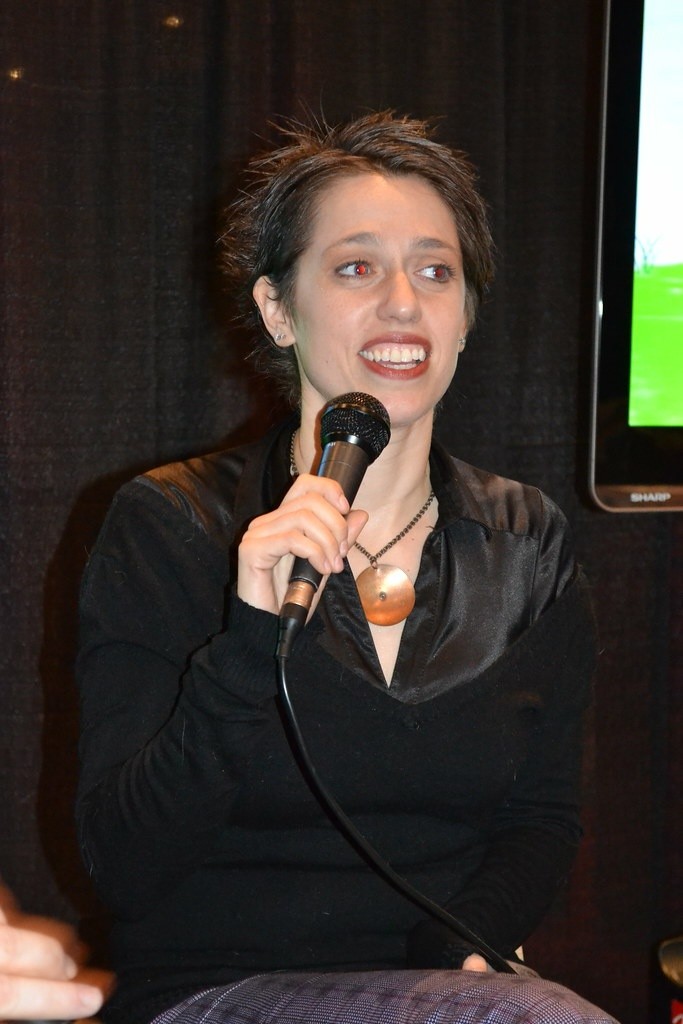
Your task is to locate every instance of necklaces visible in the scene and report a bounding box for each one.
[290,429,435,625]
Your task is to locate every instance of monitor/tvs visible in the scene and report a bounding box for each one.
[581,0,681,517]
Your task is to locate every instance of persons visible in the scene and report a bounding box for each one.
[76,114,619,1024]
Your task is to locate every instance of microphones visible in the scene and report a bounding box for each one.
[274,392,391,662]
